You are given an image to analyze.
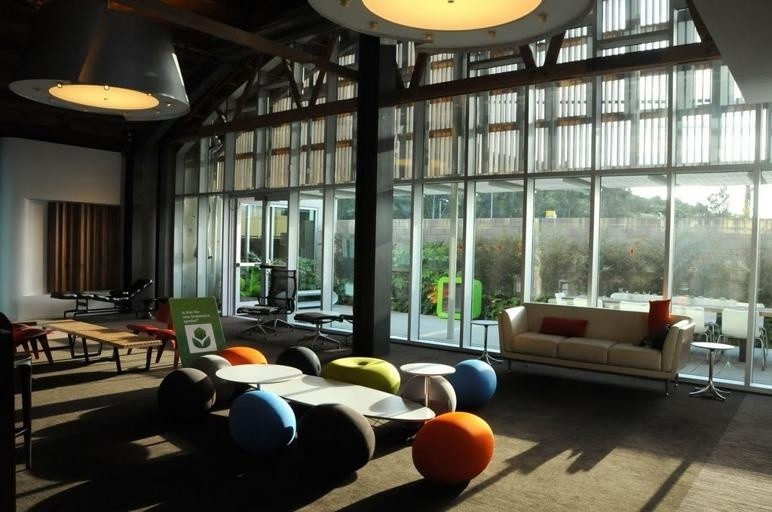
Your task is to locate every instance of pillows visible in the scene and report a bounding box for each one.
[652,320,670,349]
[558,318,588,337]
[648,300,671,338]
[540,316,563,335]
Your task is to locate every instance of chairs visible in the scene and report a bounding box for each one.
[549,293,772,384]
[156,312,183,370]
[237,264,296,341]
[51,279,153,318]
[126,303,171,354]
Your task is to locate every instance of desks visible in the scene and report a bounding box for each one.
[248,374,435,449]
[401,363,456,443]
[471,320,503,367]
[215,364,302,391]
[36,319,162,373]
[689,342,734,401]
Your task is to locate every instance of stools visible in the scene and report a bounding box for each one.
[11,353,32,469]
[339,314,353,344]
[12,322,55,366]
[293,312,343,352]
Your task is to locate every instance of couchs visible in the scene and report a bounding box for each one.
[498,302,695,396]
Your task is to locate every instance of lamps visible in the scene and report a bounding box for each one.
[308,0,598,50]
[8,3,192,123]
